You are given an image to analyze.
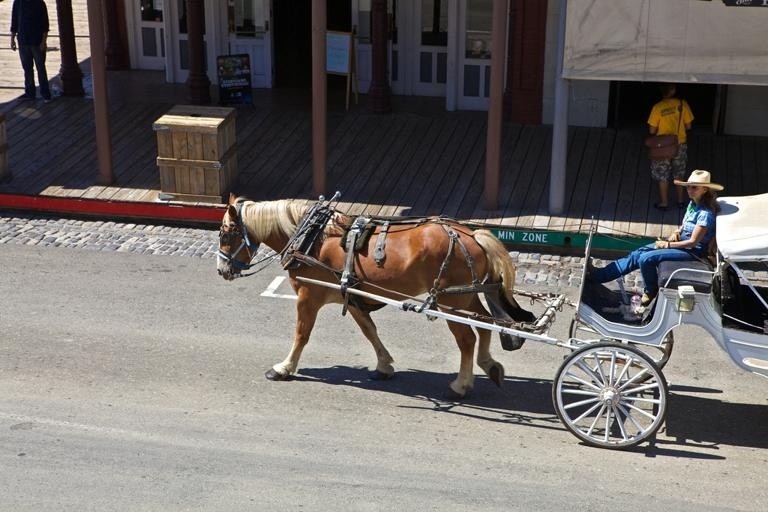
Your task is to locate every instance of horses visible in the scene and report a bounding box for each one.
[216,192,518,400]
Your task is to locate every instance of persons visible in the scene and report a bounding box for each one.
[142,1,158,21]
[647,81,695,210]
[11,0,53,104]
[586,170,725,306]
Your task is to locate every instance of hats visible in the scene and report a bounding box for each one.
[674,169,725,191]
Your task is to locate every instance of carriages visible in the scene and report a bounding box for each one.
[217,192,767,448]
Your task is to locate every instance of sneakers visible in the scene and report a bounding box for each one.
[588,256,599,286]
[654,201,686,211]
[17,94,54,103]
[640,293,653,305]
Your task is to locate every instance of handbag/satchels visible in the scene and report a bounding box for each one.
[646,134,678,160]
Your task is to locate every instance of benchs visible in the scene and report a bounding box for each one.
[657,255,714,294]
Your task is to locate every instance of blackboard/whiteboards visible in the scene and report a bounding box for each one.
[217,54,253,105]
[326,30,352,73]
